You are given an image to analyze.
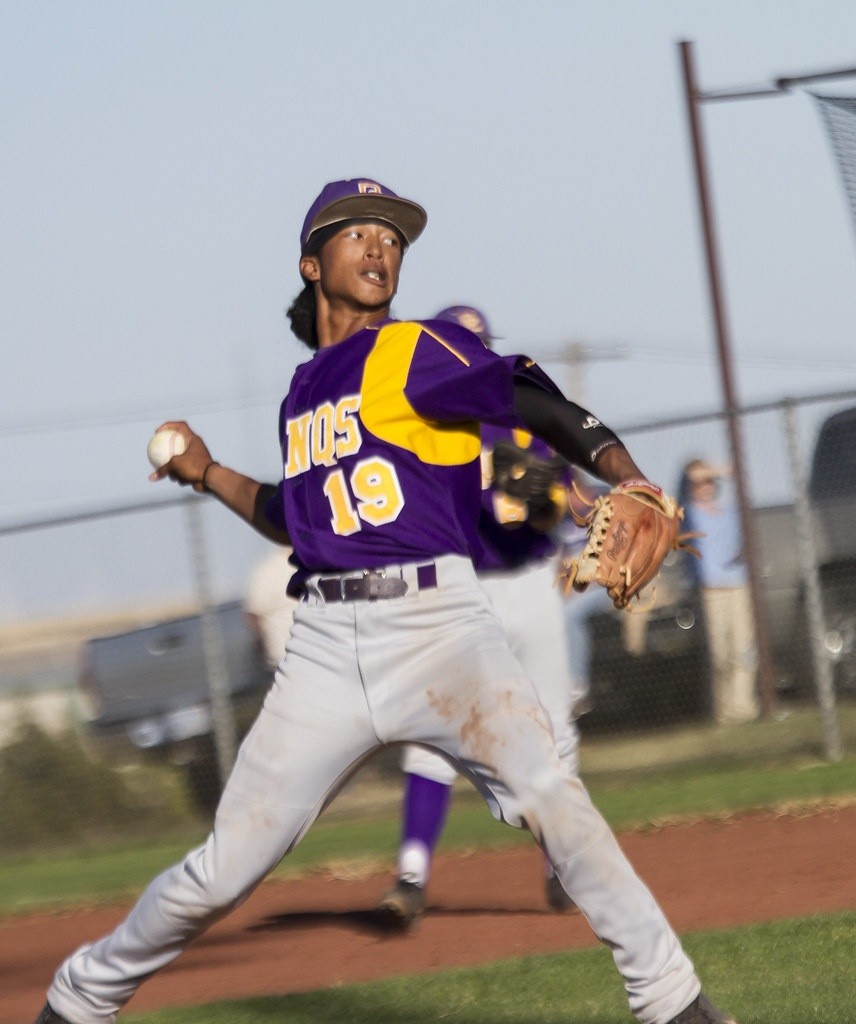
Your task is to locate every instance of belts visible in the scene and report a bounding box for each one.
[296,558,438,603]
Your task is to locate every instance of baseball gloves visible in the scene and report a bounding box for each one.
[551,481,706,612]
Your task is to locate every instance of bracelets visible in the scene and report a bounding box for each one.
[202,461,219,493]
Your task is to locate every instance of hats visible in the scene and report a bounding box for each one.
[297,178,428,255]
[431,304,505,344]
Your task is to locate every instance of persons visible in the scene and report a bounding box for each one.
[381,305,607,930]
[35,175,736,1024]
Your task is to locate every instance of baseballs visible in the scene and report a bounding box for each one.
[148,430,187,469]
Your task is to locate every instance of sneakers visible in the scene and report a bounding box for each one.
[379,879,429,927]
